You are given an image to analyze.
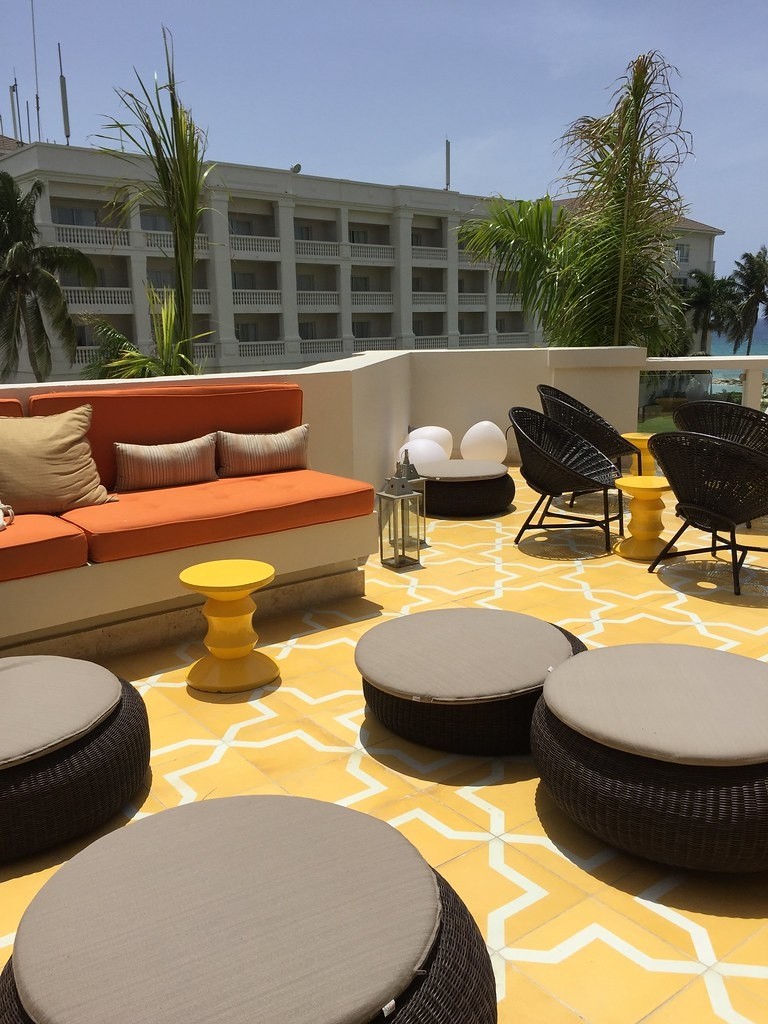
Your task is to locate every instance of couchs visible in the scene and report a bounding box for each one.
[0,380,380,662]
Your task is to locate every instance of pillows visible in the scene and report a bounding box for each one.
[217,423,312,478]
[0,404,120,517]
[112,431,219,493]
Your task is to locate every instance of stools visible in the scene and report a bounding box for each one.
[418,459,516,517]
[1,656,151,865]
[354,607,588,755]
[530,642,768,873]
[0,795,498,1024]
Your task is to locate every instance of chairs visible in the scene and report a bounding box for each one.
[672,399,768,530]
[647,431,768,595]
[536,382,642,509]
[509,405,624,552]
